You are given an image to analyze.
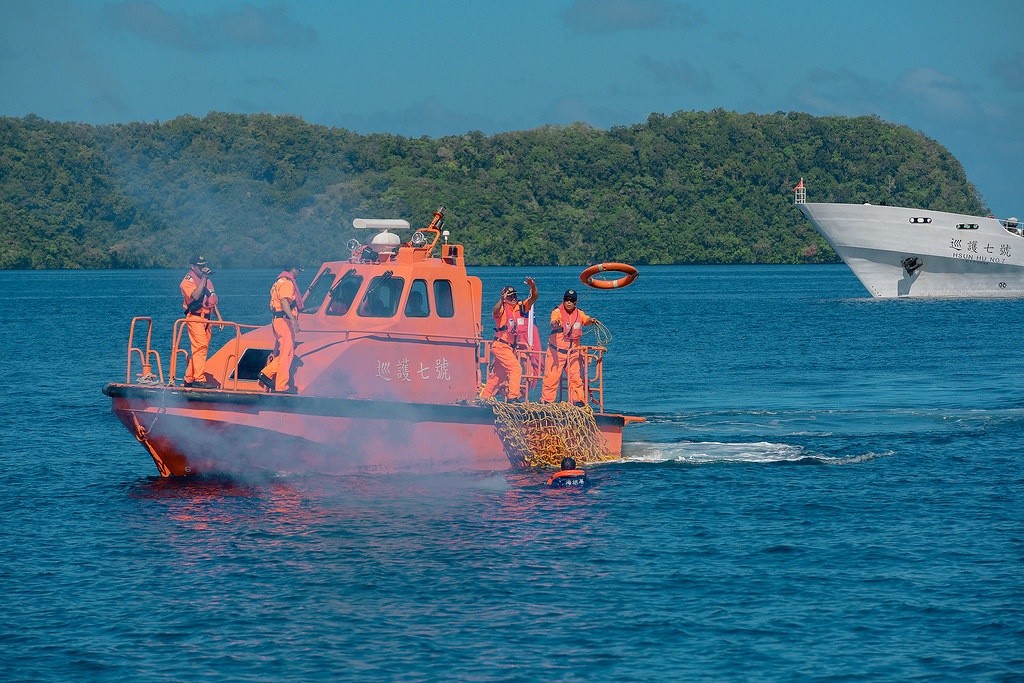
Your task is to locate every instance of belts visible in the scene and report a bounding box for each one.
[548,341,575,353]
[493,336,513,347]
[273,314,289,317]
[191,312,210,319]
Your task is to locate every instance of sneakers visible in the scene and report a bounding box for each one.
[190,380,215,389]
[183,377,193,387]
[277,386,298,394]
[256,371,274,389]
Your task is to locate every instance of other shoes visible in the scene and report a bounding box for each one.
[573,401,585,407]
[506,398,521,405]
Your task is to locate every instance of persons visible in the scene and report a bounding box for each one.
[179,254,224,389]
[405,290,428,317]
[481,276,539,406]
[258,257,303,394]
[303,282,394,317]
[540,290,596,407]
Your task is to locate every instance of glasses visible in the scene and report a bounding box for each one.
[563,299,576,303]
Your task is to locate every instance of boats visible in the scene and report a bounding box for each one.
[101,201,645,477]
[791,176,1024,299]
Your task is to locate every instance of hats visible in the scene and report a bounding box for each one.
[502,285,521,296]
[284,259,304,271]
[564,289,578,301]
[189,253,208,265]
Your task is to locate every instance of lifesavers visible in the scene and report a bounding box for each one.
[578,261,640,291]
